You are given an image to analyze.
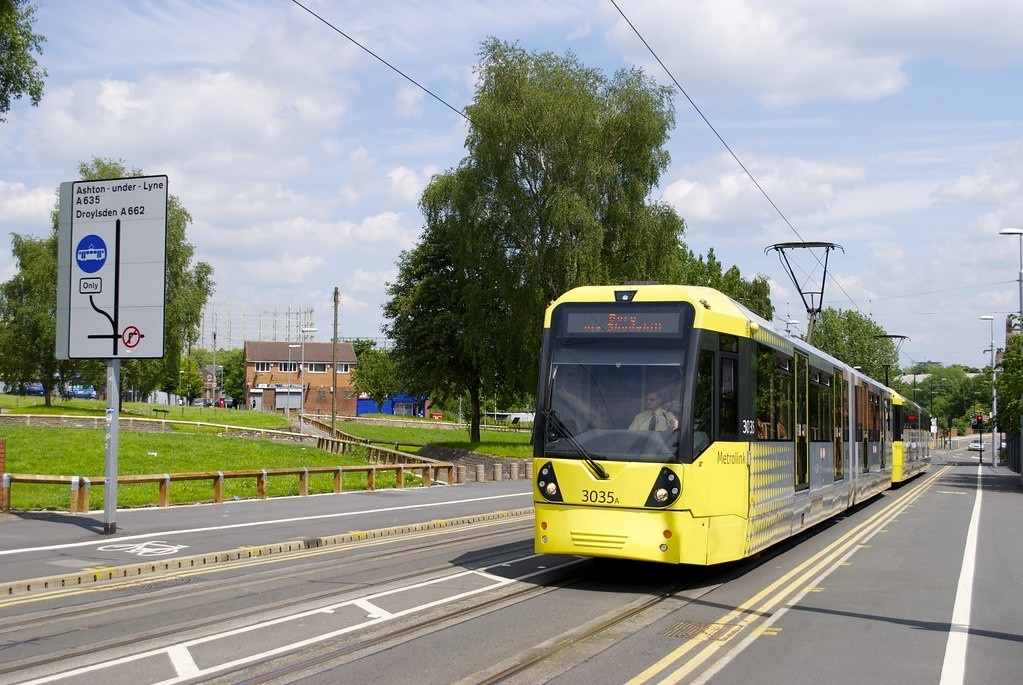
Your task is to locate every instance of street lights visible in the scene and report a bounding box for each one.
[301,327,318,435]
[218,365,223,398]
[287,344,301,420]
[980,315,997,467]
[179,370,184,395]
[999,227,1023,328]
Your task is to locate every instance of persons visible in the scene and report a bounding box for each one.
[628,390,678,432]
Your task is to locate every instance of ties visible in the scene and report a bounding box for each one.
[648,412,656,431]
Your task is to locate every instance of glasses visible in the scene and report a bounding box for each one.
[645,398,657,401]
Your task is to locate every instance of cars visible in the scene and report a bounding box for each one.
[215,398,235,408]
[6,385,43,396]
[968,439,984,452]
[192,398,210,406]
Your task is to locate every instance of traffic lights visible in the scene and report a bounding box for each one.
[977,415,981,424]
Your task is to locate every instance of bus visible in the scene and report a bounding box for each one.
[530,284,894,566]
[893,395,932,484]
